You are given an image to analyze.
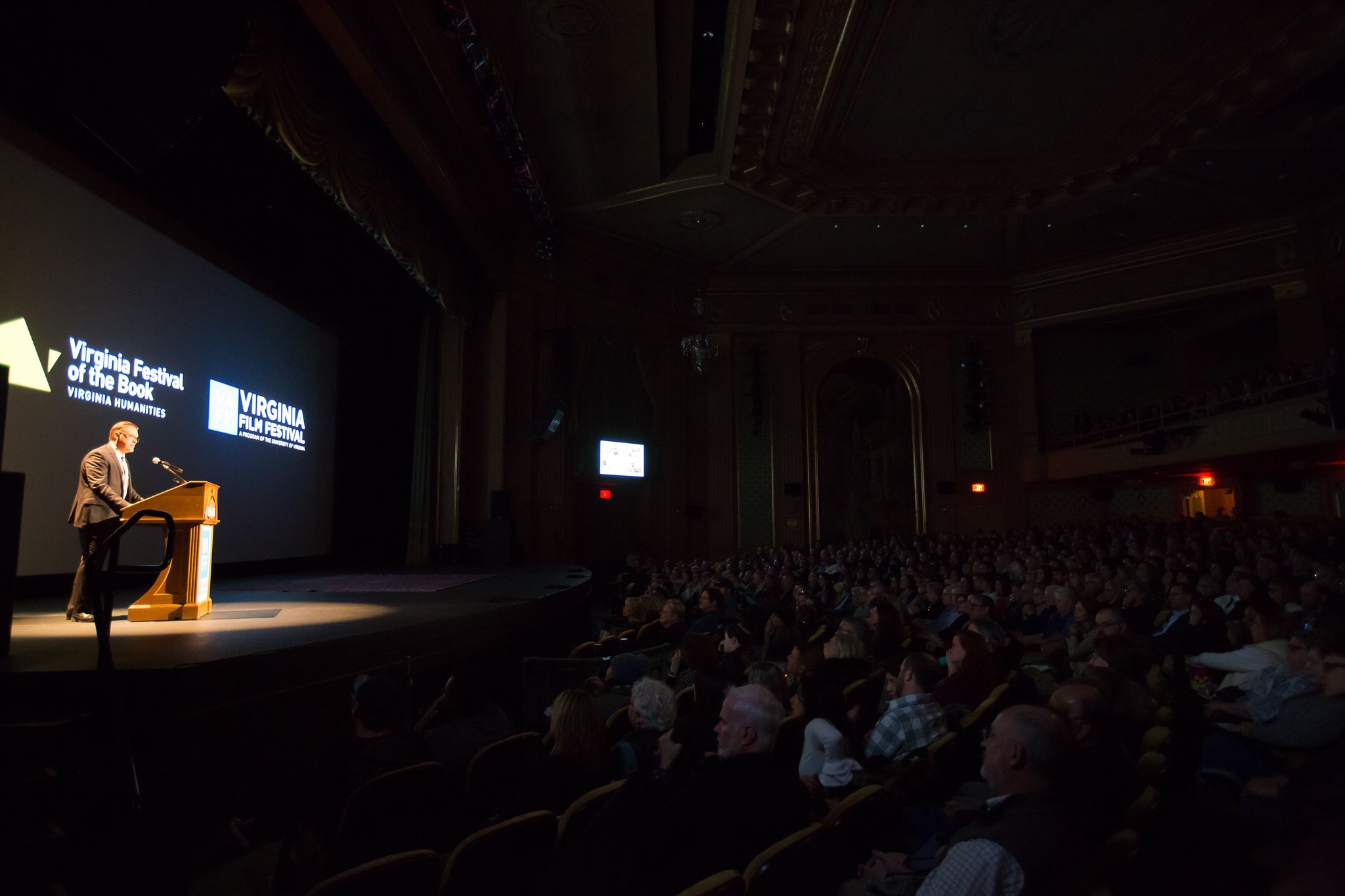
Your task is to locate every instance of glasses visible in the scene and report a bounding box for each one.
[119,432,140,442]
[950,573,958,576]
[1288,645,1304,652]
[958,600,966,604]
[699,596,710,600]
[1094,620,1121,630]
[838,627,856,634]
[1323,661,1345,673]
[968,603,984,611]
[1168,592,1187,598]
[982,728,1017,744]
[660,609,674,615]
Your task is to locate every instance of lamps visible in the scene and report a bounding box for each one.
[428,0,571,263]
[677,278,724,376]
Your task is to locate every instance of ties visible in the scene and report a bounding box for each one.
[121,456,128,500]
[1224,598,1235,609]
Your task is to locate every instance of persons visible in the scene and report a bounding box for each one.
[356,505,1345,896]
[66,421,143,622]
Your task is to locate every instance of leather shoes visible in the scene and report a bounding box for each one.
[66,611,94,622]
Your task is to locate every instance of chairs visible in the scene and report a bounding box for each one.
[217,565,1345,896]
